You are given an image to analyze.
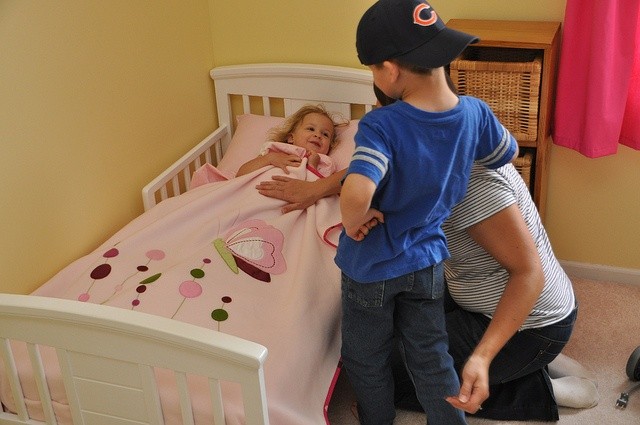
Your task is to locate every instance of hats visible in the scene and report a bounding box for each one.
[355,0,479,69]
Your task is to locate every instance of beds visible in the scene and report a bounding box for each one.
[0,61,376,424]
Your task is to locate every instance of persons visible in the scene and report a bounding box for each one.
[332,1,520,424]
[236,102,340,177]
[256,70,600,425]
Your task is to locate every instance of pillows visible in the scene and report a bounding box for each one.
[216,113,362,177]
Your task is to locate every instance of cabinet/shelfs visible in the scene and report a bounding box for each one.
[442,18,561,227]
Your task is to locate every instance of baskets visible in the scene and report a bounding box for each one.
[451,53,541,143]
[512,148,533,192]
